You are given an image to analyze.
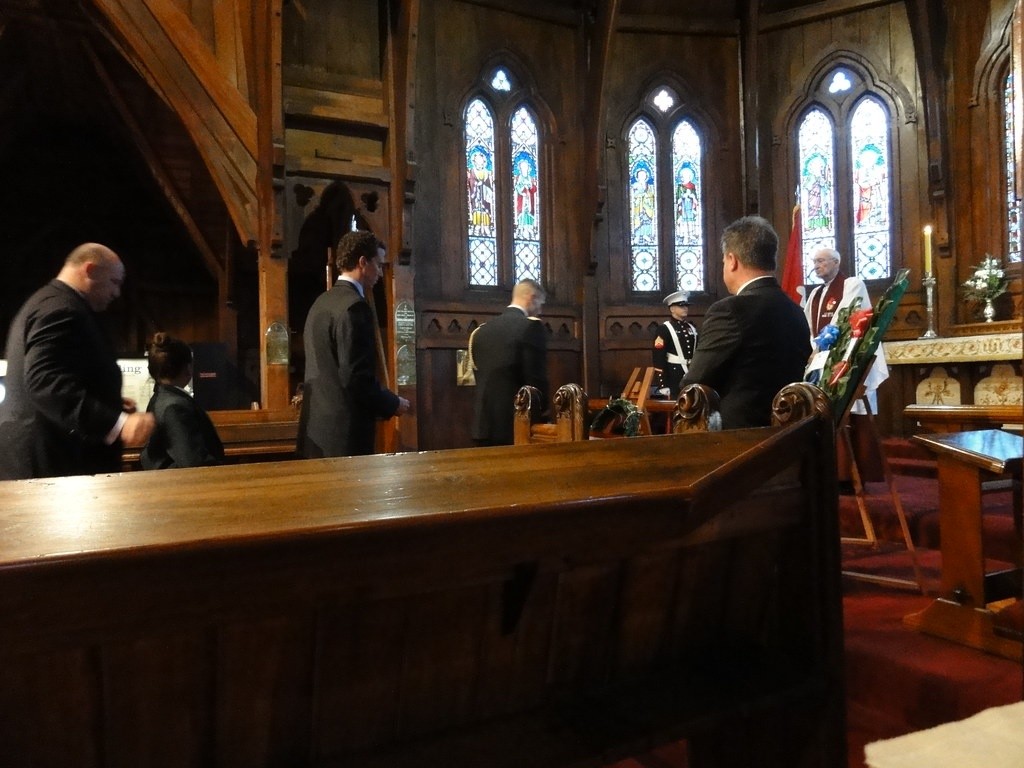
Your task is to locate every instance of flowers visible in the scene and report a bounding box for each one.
[961,252,1008,300]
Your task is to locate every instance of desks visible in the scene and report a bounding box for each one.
[0,380,848,768]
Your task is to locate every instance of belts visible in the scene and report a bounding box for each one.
[666,352,692,365]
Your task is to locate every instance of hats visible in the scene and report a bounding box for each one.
[663,290,692,307]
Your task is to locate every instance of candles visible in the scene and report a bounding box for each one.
[924,225,932,277]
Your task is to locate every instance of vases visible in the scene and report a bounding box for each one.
[982,298,996,323]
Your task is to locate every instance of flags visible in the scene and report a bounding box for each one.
[779,205,806,308]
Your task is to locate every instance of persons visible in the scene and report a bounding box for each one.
[472,279,549,445]
[678,218,813,430]
[292,231,409,459]
[137,332,224,471]
[0,243,156,482]
[653,290,699,400]
[803,247,889,496]
